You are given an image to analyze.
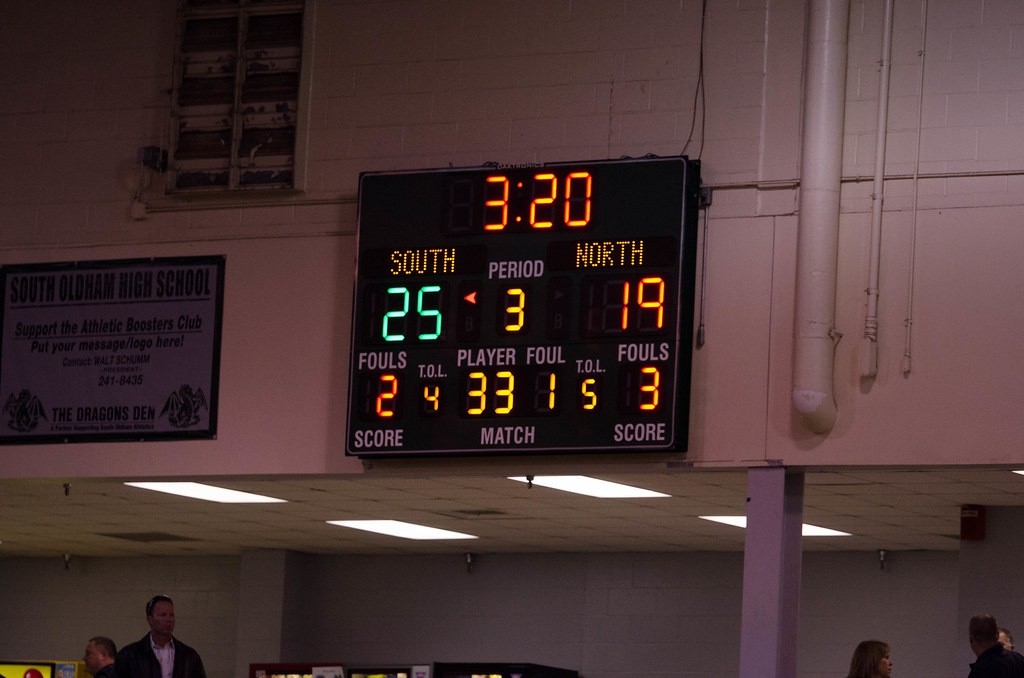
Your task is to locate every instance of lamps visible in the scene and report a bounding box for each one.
[63,554,73,570]
[465,553,473,573]
[879,549,888,569]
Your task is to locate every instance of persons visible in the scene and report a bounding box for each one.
[84,595,206,678]
[968,614,1024,678]
[848,640,893,678]
